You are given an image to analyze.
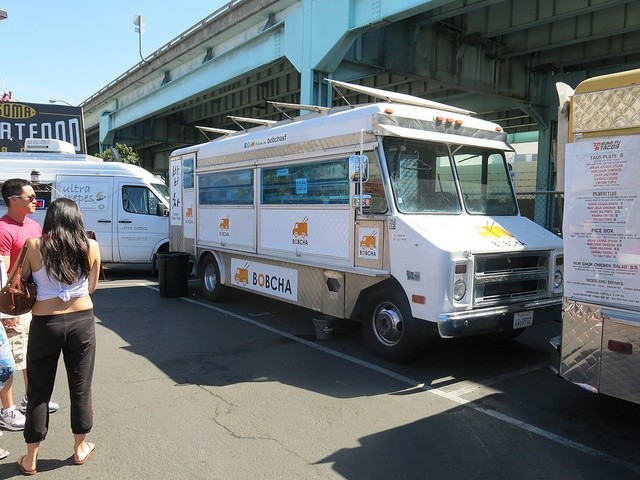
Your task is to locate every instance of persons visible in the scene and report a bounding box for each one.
[0,178,59,431]
[17,198,101,474]
[0,254,15,459]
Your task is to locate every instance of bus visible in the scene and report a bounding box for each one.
[556,67,639,410]
[169,77,564,364]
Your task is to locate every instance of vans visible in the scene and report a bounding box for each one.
[0,137,196,282]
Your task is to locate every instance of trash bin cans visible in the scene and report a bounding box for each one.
[156,251,191,297]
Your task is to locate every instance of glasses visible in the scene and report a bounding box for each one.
[8,194,36,202]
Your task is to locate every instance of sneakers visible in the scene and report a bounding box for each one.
[19,395,59,413]
[0,449,10,460]
[0,405,28,431]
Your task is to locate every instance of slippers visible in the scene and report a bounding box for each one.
[74,442,96,465]
[16,454,38,475]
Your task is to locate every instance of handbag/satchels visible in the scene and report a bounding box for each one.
[0,238,37,316]
